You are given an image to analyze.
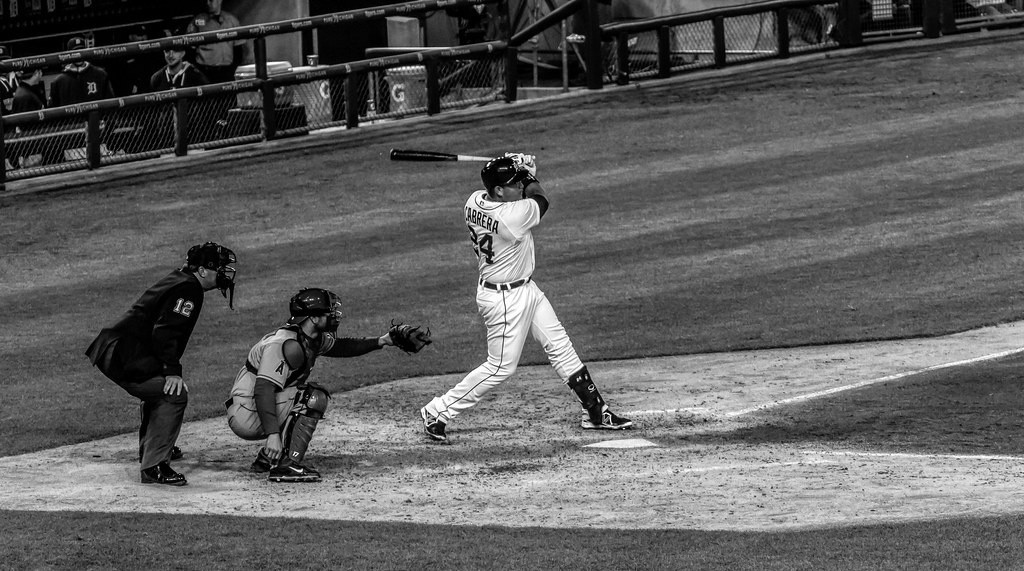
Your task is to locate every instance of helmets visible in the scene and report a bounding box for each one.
[287,288,331,324]
[482,156,528,187]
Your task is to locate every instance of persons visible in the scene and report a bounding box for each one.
[85,241,235,487]
[224,289,432,483]
[0,0,252,166]
[419,152,632,440]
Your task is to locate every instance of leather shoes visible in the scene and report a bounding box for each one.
[171,446,182,460]
[141,463,187,485]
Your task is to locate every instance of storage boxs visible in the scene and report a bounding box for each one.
[384,66,427,112]
[225,61,332,119]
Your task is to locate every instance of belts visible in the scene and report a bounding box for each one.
[480,276,532,290]
[225,398,234,409]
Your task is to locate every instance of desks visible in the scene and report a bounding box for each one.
[226,104,308,144]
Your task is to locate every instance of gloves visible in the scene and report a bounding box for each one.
[505,152,536,177]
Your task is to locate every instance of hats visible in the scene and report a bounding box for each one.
[186,242,221,269]
[68,38,86,49]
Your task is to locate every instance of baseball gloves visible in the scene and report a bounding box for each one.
[388,317,433,357]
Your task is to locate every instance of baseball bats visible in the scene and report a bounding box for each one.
[388,148,535,168]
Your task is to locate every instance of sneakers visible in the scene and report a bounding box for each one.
[421,407,446,440]
[251,448,278,472]
[581,405,632,431]
[270,463,321,482]
